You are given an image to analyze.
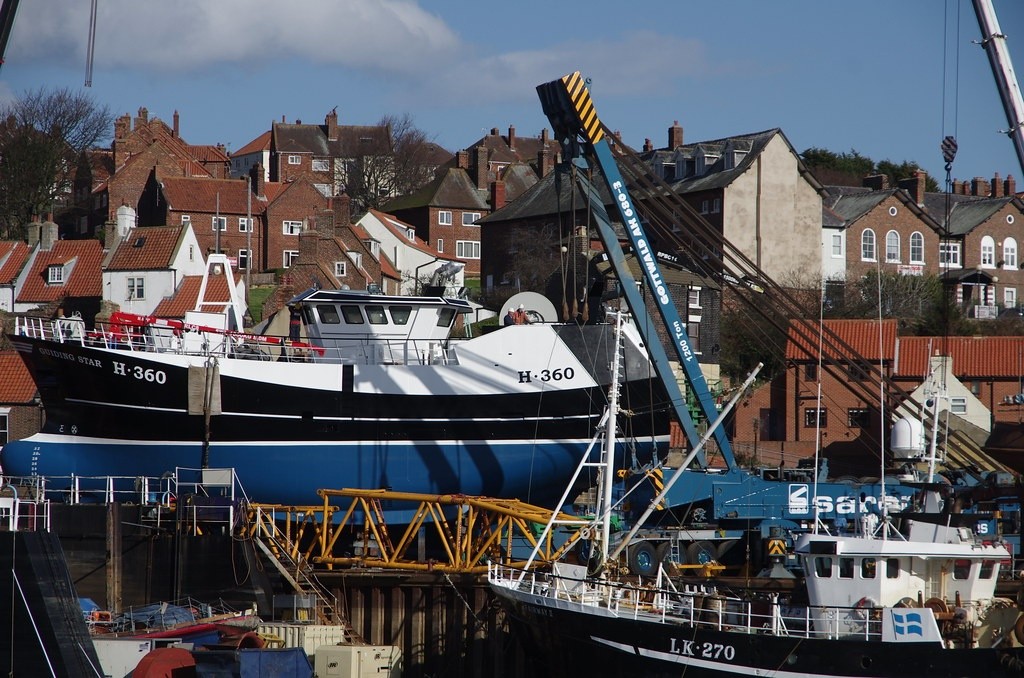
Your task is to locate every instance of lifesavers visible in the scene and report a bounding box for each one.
[854,596,879,623]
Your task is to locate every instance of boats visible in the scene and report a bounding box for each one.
[2,1,1024,676]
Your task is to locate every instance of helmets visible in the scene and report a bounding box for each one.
[508,308,515,313]
[518,305,524,308]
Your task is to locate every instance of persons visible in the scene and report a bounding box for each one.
[54,306,86,345]
[512,304,532,325]
[504,308,518,327]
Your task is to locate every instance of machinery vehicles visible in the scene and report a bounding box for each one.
[935,0,1024,389]
